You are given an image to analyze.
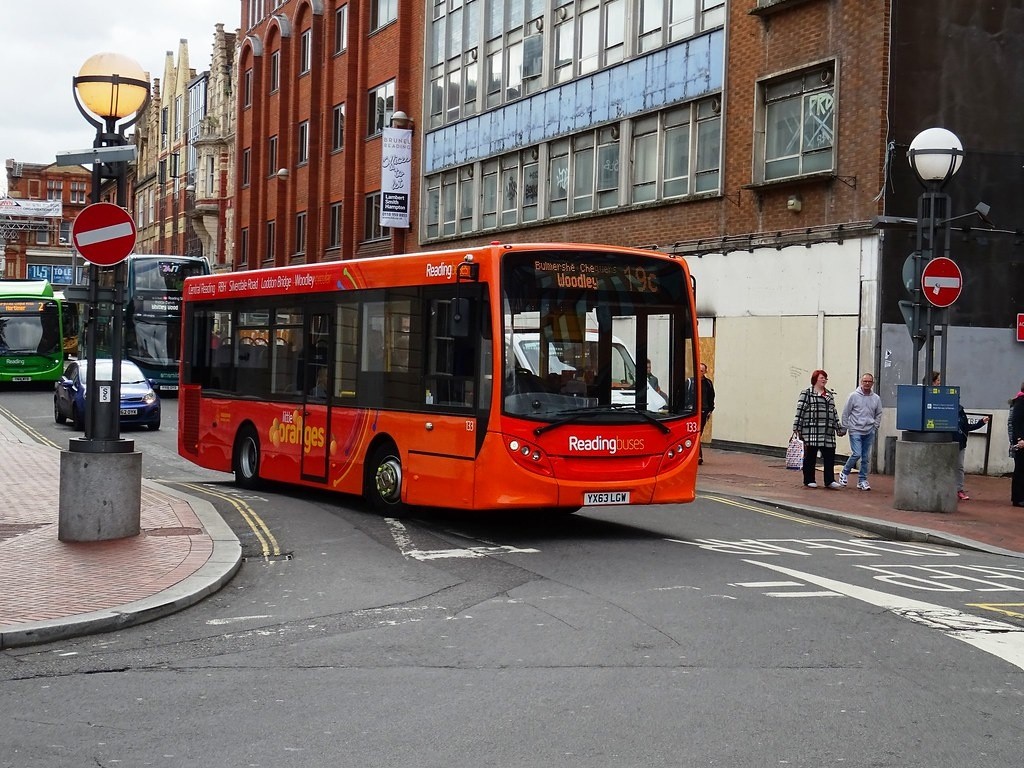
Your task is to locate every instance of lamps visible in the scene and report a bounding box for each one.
[277,168,290,180]
[185,186,195,194]
[391,110,414,126]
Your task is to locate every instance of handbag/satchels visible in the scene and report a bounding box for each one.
[786,430,804,470]
[700,413,713,444]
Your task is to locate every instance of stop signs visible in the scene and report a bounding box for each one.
[921,257,962,307]
[72,201,137,268]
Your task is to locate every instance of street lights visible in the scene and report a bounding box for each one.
[893,127,966,513]
[58,52,152,541]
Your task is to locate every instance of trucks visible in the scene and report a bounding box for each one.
[506,312,667,411]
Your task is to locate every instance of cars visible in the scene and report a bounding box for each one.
[54,359,161,431]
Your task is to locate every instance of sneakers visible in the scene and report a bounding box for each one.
[839,471,848,486]
[857,480,871,491]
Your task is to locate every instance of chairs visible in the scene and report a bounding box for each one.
[217,336,289,369]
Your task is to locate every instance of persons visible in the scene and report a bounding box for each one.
[793,370,843,488]
[1007,383,1024,507]
[647,359,658,391]
[311,368,327,397]
[686,363,715,464]
[839,373,882,490]
[922,371,940,385]
[391,336,409,372]
[954,404,989,500]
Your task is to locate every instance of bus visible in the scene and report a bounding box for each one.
[0,252,212,399]
[178,241,701,520]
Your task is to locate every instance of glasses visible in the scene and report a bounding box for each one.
[863,381,872,383]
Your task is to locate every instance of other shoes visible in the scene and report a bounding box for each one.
[957,490,969,500]
[699,458,704,465]
[824,482,841,489]
[1012,501,1024,507]
[807,482,818,488]
